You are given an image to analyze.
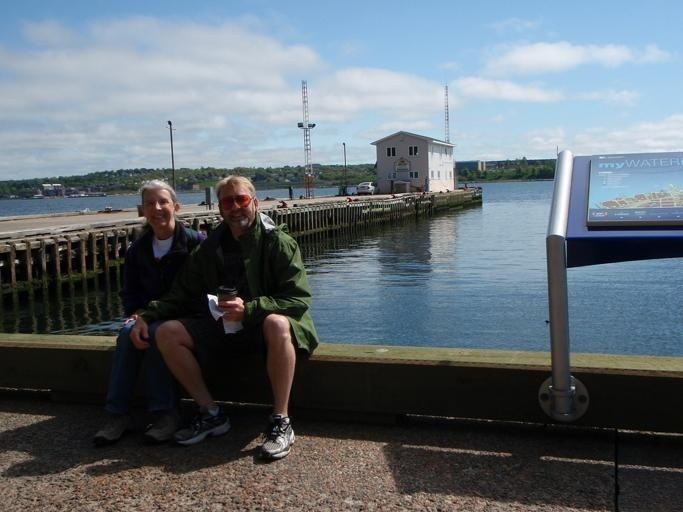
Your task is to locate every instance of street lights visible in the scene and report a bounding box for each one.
[342,143,346,184]
[296,122,316,197]
[166,121,176,192]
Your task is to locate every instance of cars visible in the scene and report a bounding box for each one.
[356,181,379,195]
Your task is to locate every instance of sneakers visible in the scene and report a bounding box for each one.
[143,412,186,442]
[91,413,137,444]
[172,404,231,445]
[261,412,296,460]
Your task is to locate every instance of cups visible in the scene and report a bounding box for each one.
[217,284,238,314]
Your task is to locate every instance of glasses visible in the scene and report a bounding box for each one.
[219,195,254,211]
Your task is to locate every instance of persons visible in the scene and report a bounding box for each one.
[126,174,318,461]
[91,178,209,446]
